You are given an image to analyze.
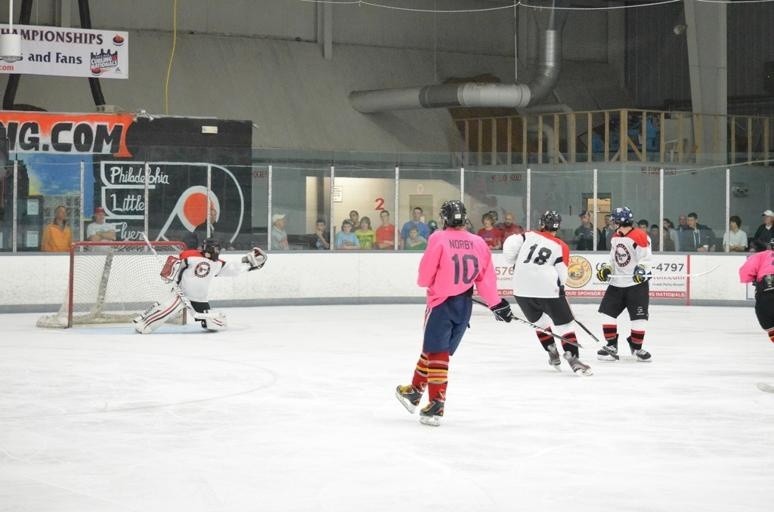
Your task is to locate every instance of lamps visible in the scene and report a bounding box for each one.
[1,0,22,63]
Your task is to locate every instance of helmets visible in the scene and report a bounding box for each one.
[202,239,221,262]
[537,211,561,232]
[437,200,466,231]
[767,238,774,249]
[607,207,633,233]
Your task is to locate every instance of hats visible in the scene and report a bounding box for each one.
[343,219,353,226]
[762,209,773,217]
[272,214,285,224]
[579,211,590,216]
[429,221,436,229]
[94,207,104,213]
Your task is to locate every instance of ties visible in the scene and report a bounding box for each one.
[694,231,699,250]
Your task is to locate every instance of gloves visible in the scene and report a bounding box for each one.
[490,298,512,322]
[632,264,645,282]
[596,266,611,281]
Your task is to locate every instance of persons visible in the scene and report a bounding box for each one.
[188,209,226,254]
[721,214,749,251]
[308,207,525,249]
[392,201,516,423]
[503,209,593,378]
[754,210,773,252]
[569,210,716,252]
[84,204,117,253]
[133,238,226,337]
[132,231,225,323]
[579,205,656,365]
[267,212,293,251]
[35,204,73,254]
[737,242,774,346]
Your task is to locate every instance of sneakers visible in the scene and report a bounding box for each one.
[627,336,650,359]
[563,350,590,373]
[420,390,445,416]
[547,343,560,365]
[396,382,427,406]
[598,334,618,355]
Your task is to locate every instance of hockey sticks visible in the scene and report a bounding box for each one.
[573,318,637,361]
[607,264,720,278]
[471,297,598,368]
[137,232,210,320]
